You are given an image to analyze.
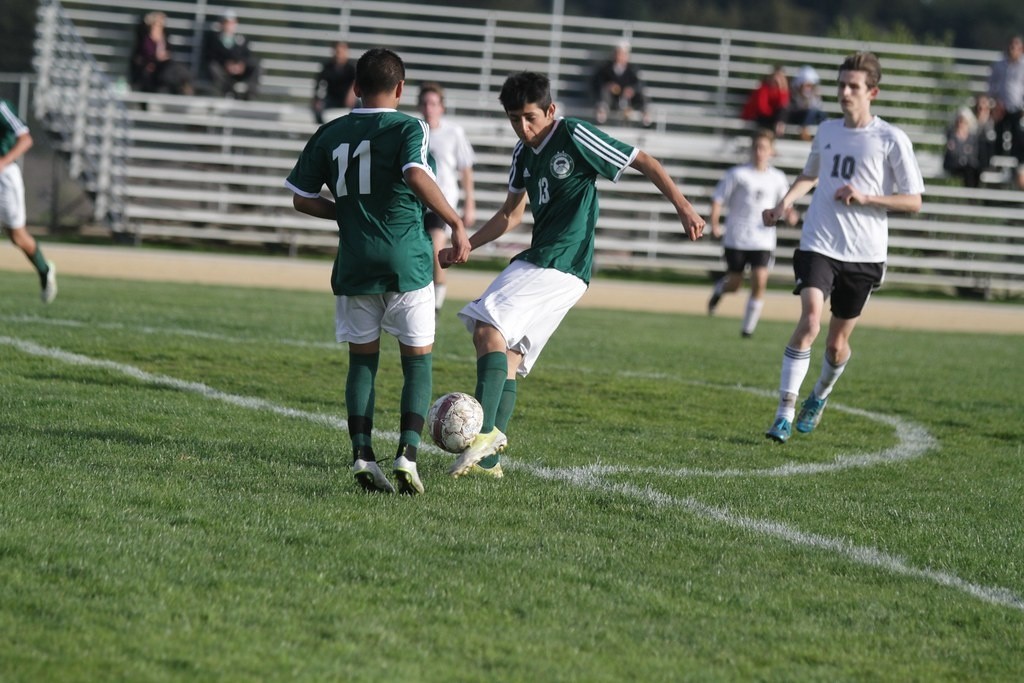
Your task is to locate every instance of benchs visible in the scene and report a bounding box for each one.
[31,0,1024,296]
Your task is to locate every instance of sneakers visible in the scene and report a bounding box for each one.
[448,426,508,479]
[796,390,828,433]
[353,458,395,493]
[765,417,792,444]
[471,462,504,479]
[395,457,425,496]
[41,263,58,304]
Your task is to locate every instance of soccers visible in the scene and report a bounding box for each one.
[426,391,484,453]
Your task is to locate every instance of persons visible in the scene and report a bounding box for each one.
[131,11,184,109]
[742,64,828,137]
[203,13,257,101]
[708,130,798,337]
[943,34,1024,187]
[438,69,706,483]
[0,95,57,303]
[590,41,651,127]
[416,87,476,311]
[311,43,360,125]
[285,49,471,495]
[766,52,926,441]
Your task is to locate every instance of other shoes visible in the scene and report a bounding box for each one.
[708,294,720,311]
[743,331,752,339]
